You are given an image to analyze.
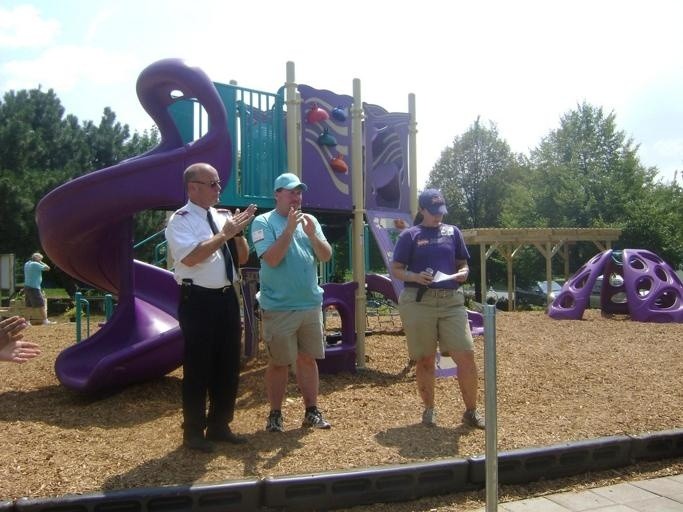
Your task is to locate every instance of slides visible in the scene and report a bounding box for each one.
[33,56,232,393]
[364,273,484,336]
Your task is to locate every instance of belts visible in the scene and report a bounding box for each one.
[191,285,234,295]
[423,288,457,298]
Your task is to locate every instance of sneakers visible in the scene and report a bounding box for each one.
[302,409,331,429]
[422,408,433,427]
[463,410,485,429]
[266,414,283,432]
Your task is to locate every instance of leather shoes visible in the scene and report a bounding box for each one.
[206,423,248,444]
[183,424,214,453]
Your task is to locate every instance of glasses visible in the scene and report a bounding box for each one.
[192,180,221,188]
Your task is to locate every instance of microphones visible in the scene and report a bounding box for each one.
[415,268,434,302]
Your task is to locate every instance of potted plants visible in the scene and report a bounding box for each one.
[10,292,47,324]
[64,307,86,322]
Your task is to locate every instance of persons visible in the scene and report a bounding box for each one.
[165,162,258,454]
[251,173,332,433]
[0,316,41,364]
[24,253,57,327]
[390,189,485,429]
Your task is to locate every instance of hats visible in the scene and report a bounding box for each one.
[416,187,448,216]
[274,173,308,193]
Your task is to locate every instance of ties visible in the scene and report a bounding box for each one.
[207,211,233,284]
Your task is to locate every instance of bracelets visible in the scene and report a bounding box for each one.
[235,231,244,237]
